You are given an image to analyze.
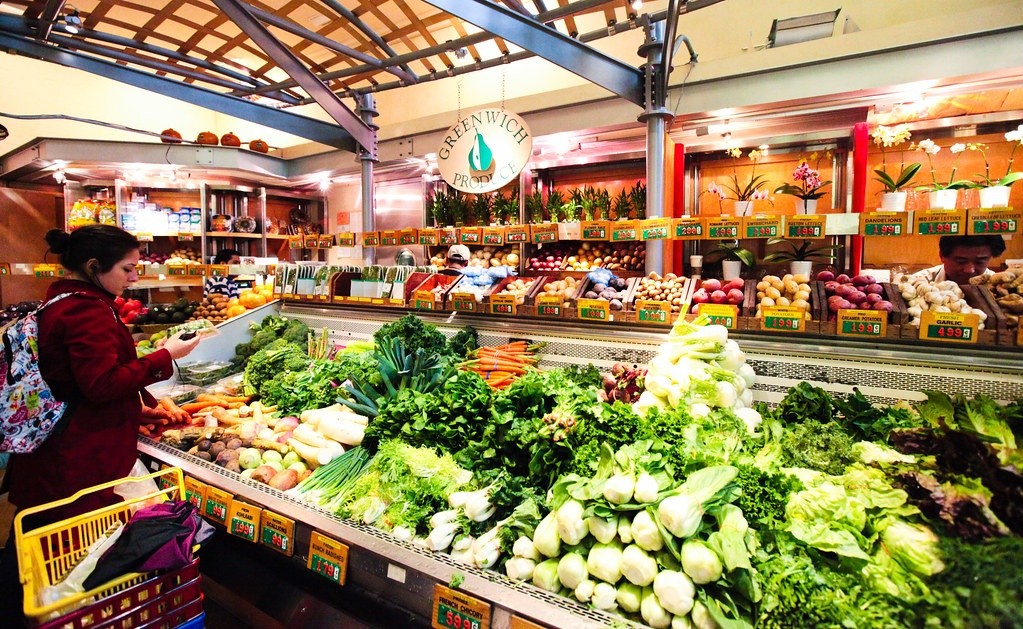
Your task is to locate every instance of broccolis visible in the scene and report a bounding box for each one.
[228,323,316,375]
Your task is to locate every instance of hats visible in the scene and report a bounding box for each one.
[447,244,471,261]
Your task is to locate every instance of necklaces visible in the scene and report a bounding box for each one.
[449,266,459,269]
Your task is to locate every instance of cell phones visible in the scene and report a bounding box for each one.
[182,330,196,341]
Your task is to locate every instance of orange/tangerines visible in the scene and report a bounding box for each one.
[227,284,273,319]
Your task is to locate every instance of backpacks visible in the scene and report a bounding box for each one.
[0,291,117,454]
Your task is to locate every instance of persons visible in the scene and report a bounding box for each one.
[202,249,240,301]
[0,225,200,629]
[911,235,1006,285]
[437,244,471,276]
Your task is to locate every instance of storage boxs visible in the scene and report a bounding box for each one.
[121,192,203,235]
[274,271,1023,348]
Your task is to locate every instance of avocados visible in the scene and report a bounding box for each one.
[135,299,199,324]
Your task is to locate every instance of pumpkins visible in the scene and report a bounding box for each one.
[222,132,240,147]
[197,131,218,145]
[249,139,268,153]
[161,128,182,143]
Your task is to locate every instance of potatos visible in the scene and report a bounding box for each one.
[755,274,813,320]
[585,278,632,310]
[819,271,893,321]
[192,435,263,478]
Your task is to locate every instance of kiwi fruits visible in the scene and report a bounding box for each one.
[190,294,228,320]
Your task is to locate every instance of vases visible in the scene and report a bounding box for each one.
[734,202,752,217]
[796,199,817,215]
[930,189,958,209]
[883,192,907,211]
[979,185,1011,207]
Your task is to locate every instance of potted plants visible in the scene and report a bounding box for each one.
[526,182,648,221]
[426,188,517,227]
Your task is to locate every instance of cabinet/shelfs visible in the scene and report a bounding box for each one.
[62,182,328,331]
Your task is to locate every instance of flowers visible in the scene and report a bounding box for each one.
[958,125,1023,190]
[907,138,980,193]
[871,124,921,196]
[708,142,774,216]
[773,147,837,214]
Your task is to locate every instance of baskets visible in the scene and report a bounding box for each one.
[13,466,208,629]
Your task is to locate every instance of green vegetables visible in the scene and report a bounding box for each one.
[241,316,1023,629]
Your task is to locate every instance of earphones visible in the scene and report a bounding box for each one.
[90,264,98,273]
